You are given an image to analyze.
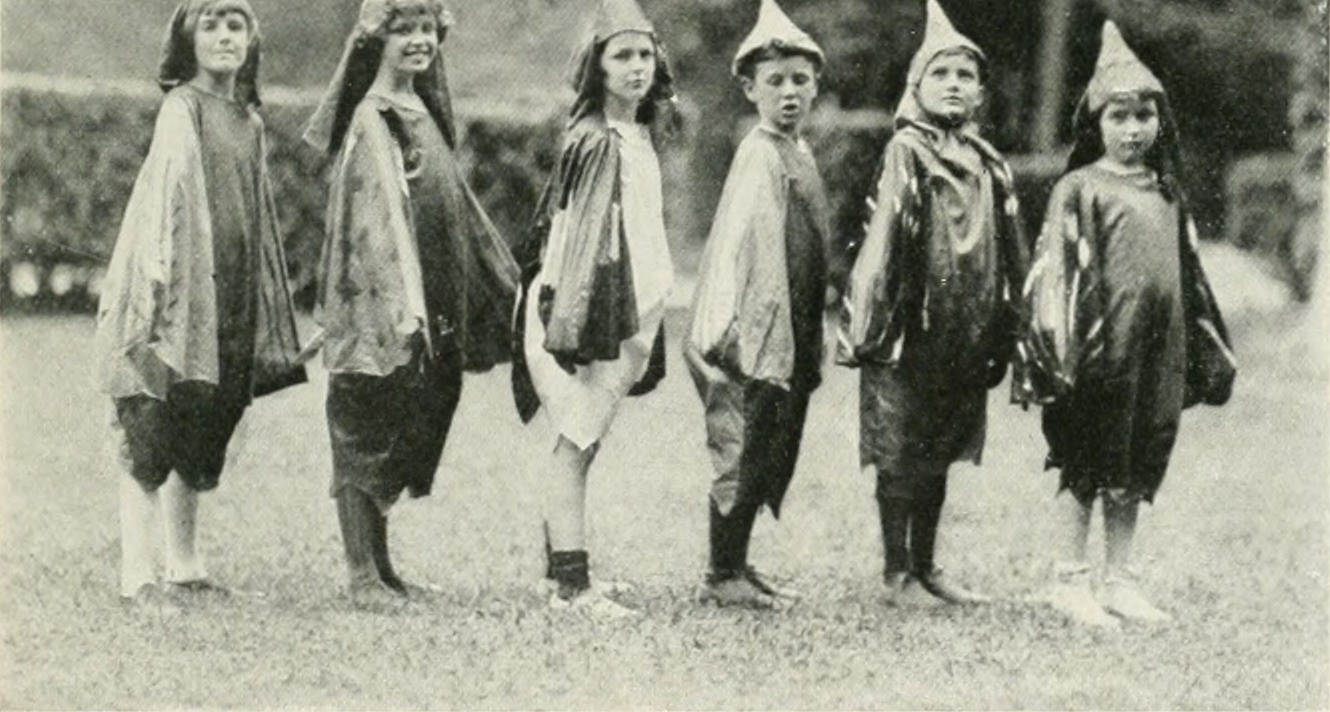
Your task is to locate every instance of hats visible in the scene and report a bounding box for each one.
[730,0,827,78]
[895,0,987,131]
[565,0,654,130]
[1065,16,1164,170]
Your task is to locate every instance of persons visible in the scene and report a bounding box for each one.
[1014,18,1241,580]
[511,0,673,616]
[679,0,833,610]
[93,0,310,604]
[834,0,1033,609]
[300,0,524,616]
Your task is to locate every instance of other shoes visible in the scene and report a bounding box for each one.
[880,570,945,609]
[168,575,267,601]
[537,575,631,600]
[925,562,992,606]
[1049,582,1170,635]
[695,570,803,608]
[344,575,443,616]
[550,590,639,619]
[120,580,180,614]
